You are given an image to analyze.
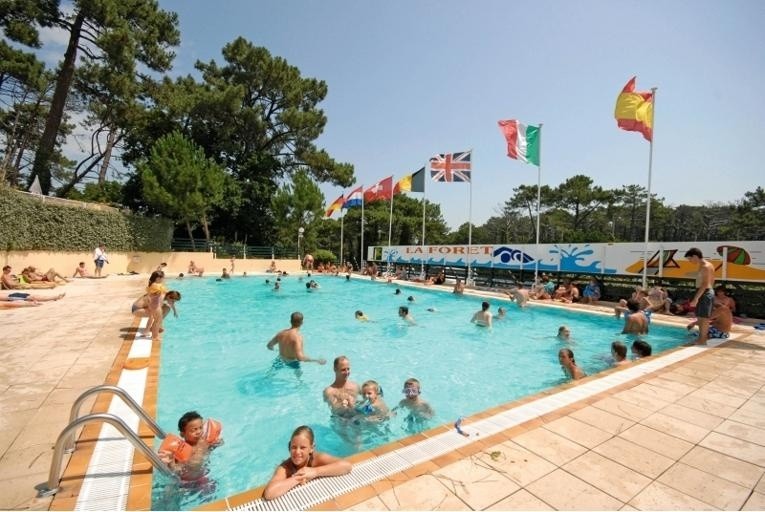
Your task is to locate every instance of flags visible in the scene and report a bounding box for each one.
[393,167,424,196]
[326,194,343,218]
[428,151,471,183]
[613,75,654,141]
[341,186,362,213]
[364,175,392,204]
[497,118,542,167]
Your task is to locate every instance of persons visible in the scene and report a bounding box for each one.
[264,311,328,380]
[131,262,181,346]
[177,253,355,291]
[558,348,587,385]
[322,356,392,453]
[354,260,604,346]
[0,241,110,309]
[158,410,225,503]
[263,425,352,501]
[608,247,737,368]
[392,378,435,434]
